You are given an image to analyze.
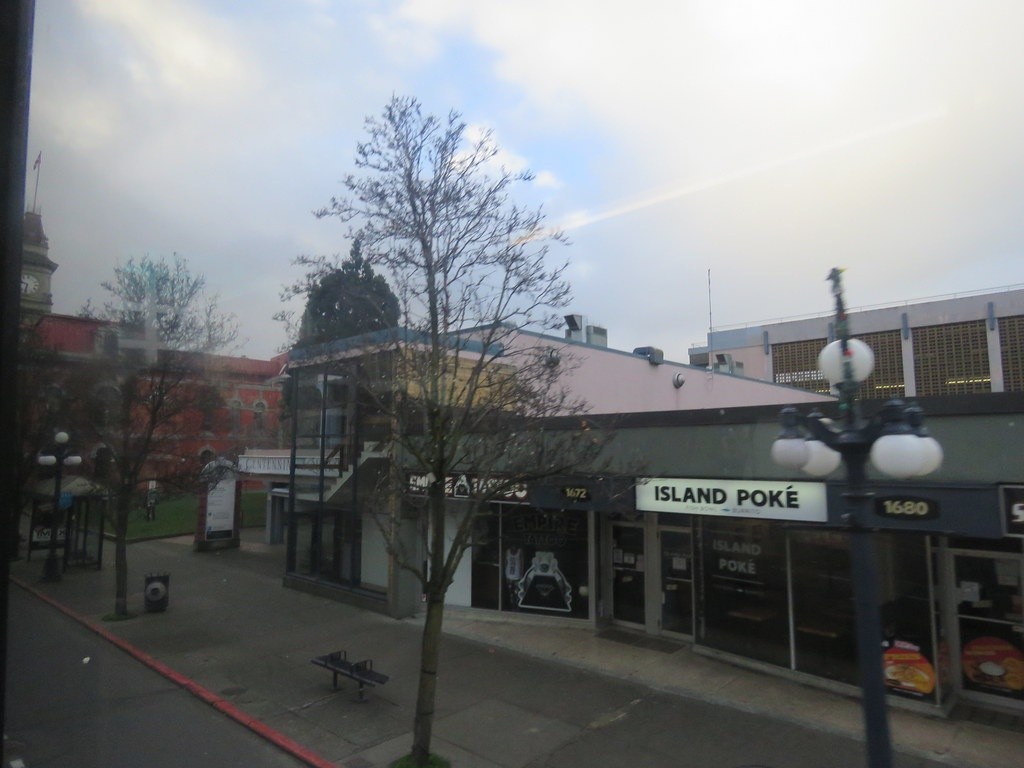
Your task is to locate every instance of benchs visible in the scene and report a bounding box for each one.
[312,649,389,703]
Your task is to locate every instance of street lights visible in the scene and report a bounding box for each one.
[38,431,83,583]
[773,268,943,768]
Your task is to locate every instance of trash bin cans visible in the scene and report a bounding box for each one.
[144,571,171,613]
